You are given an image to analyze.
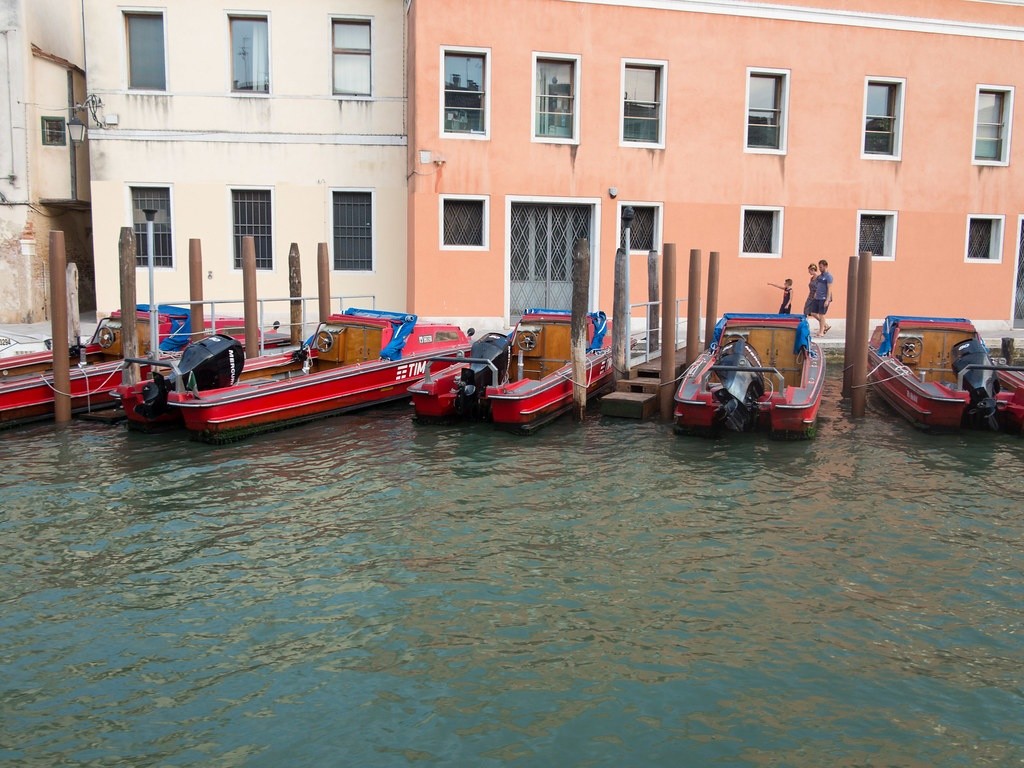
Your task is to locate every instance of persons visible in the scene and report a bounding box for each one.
[804,260,833,338]
[767,279,793,314]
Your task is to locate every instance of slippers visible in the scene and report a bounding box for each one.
[811,333,823,337]
[824,326,831,334]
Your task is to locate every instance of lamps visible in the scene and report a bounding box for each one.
[68,109,88,149]
[436,160,446,168]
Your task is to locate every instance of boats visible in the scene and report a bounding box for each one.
[672,310,826,441]
[0,299,288,430]
[406,306,640,437]
[107,306,476,446]
[867,315,1024,435]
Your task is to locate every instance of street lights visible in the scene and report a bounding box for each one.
[621,205,638,372]
[141,207,158,372]
